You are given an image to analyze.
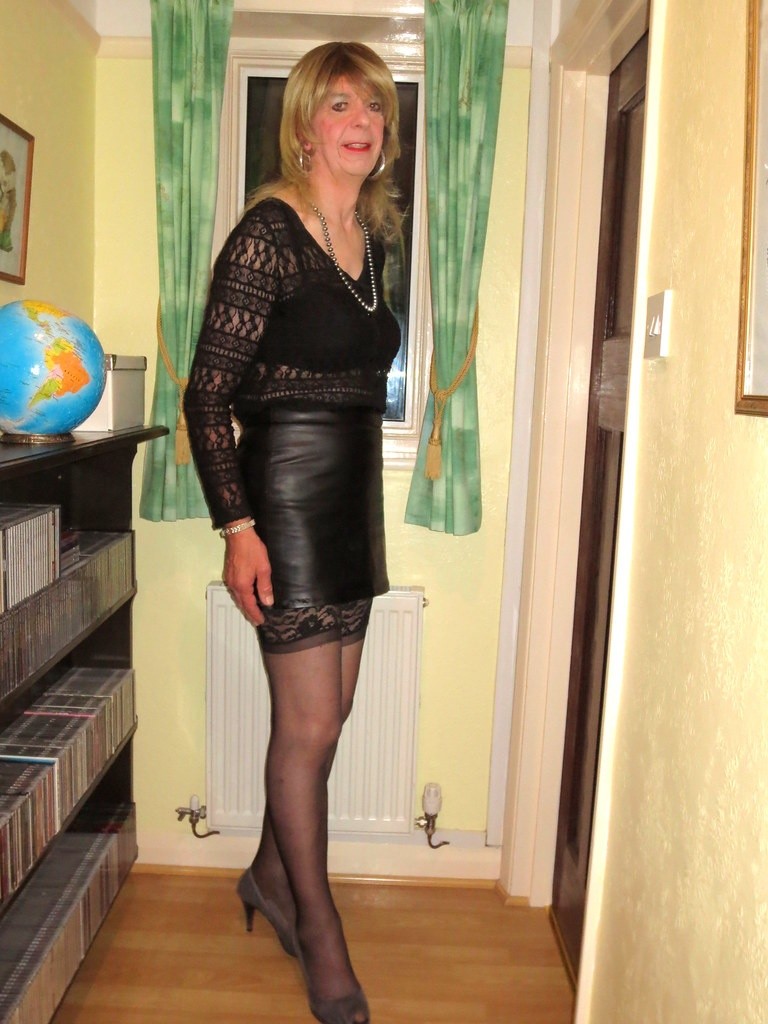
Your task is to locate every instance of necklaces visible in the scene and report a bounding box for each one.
[307,201,380,314]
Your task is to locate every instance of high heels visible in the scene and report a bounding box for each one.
[236,868,298,956]
[300,927,370,1024]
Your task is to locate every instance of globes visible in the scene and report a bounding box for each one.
[0,299,107,444]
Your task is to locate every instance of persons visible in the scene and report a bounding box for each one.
[184,42,405,1024]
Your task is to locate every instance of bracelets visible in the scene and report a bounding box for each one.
[220,519,256,537]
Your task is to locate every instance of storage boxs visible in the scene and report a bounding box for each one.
[73,353,148,432]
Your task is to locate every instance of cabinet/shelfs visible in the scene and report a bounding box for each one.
[0,425,170,1024]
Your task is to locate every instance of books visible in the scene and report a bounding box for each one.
[1,504,136,1024]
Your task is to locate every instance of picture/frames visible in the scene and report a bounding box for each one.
[732,0,768,417]
[0,113,35,285]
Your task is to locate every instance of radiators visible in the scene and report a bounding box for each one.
[175,580,451,849]
